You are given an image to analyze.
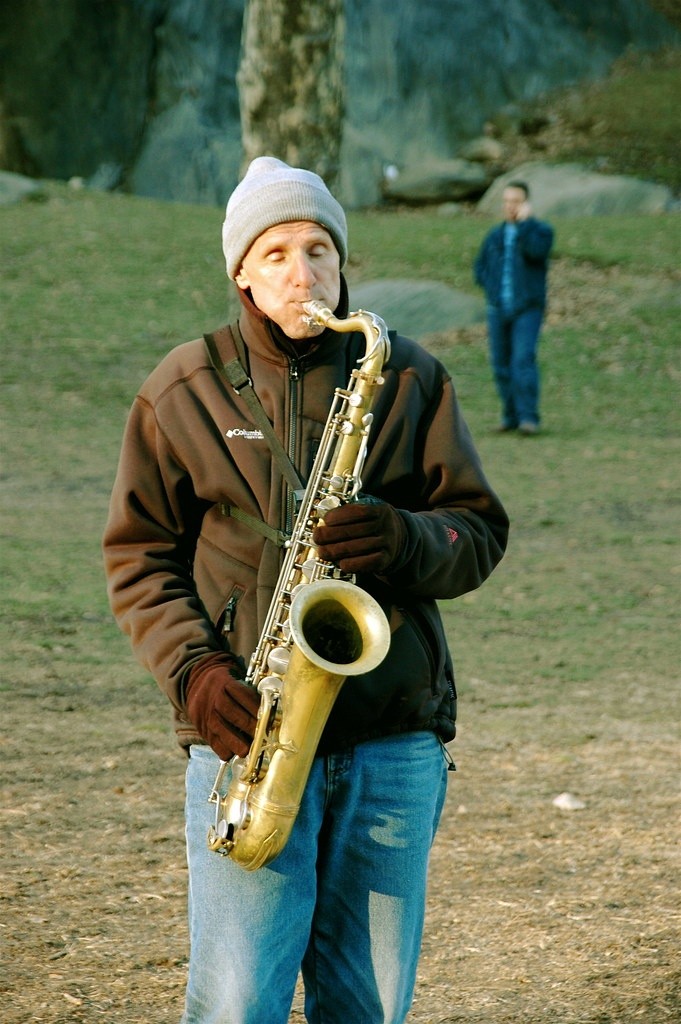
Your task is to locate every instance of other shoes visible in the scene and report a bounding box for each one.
[520,422,536,432]
[496,422,519,433]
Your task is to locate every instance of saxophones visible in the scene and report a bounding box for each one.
[203,298,394,872]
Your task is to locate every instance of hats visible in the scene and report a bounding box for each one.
[222,156,347,282]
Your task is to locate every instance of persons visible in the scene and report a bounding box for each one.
[473,181,552,435]
[103,157,510,1023]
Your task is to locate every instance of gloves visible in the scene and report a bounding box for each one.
[185,650,273,761]
[311,491,407,576]
